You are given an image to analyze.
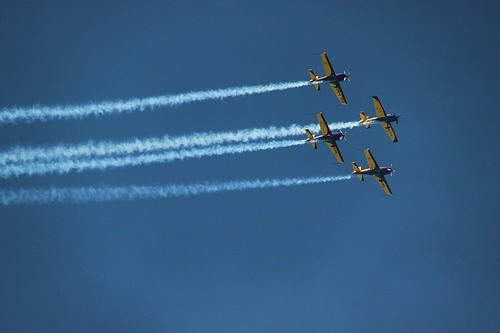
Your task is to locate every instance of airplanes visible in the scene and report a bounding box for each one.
[359,95,401,143]
[305,112,346,164]
[308,52,350,105]
[351,148,396,196]
